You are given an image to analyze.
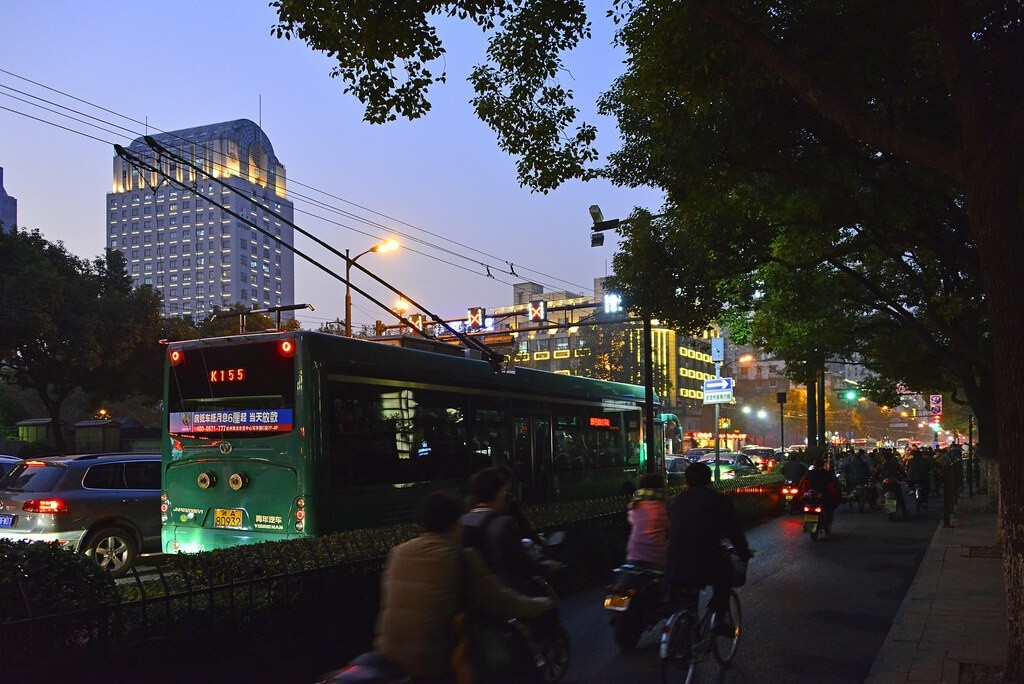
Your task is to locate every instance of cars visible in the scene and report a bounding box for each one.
[827,437,974,458]
[695,452,762,483]
[741,447,776,472]
[682,445,808,464]
[664,453,694,488]
[767,451,798,474]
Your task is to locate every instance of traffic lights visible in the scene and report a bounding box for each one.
[836,390,858,400]
[932,423,940,434]
[603,291,626,316]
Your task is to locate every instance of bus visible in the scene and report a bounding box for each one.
[158,329,684,572]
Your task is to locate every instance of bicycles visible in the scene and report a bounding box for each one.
[658,547,758,684]
[906,471,926,513]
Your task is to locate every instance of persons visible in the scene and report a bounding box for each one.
[781,442,978,522]
[455,463,546,591]
[372,490,554,684]
[626,473,670,566]
[666,462,749,664]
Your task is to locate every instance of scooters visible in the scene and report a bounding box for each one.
[782,478,800,517]
[603,560,684,650]
[314,527,584,684]
[801,464,910,542]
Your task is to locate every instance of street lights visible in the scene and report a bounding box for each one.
[714,353,753,482]
[742,405,768,447]
[345,236,401,337]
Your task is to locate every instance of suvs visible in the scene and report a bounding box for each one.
[0,449,159,580]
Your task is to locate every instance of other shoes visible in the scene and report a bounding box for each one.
[714,624,736,639]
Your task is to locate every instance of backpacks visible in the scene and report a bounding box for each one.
[460,513,509,550]
